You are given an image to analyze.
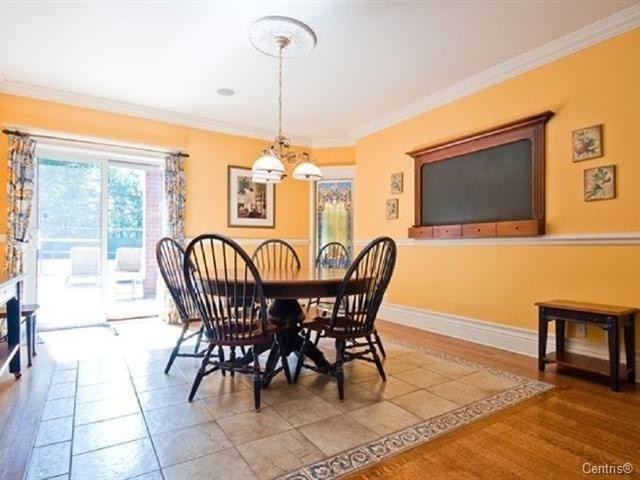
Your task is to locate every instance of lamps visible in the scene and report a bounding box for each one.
[247,15,323,183]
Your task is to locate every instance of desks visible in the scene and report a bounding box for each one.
[0,270,25,380]
[534,299,640,391]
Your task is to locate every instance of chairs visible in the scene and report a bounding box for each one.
[182,233,291,409]
[65,247,99,288]
[302,241,349,325]
[250,239,300,270]
[293,235,397,400]
[156,237,248,377]
[111,248,143,298]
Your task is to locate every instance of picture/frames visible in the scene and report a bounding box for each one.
[391,172,403,194]
[227,165,276,228]
[388,198,398,216]
[572,124,603,162]
[583,165,616,202]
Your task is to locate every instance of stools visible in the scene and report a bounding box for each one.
[0,303,40,368]
